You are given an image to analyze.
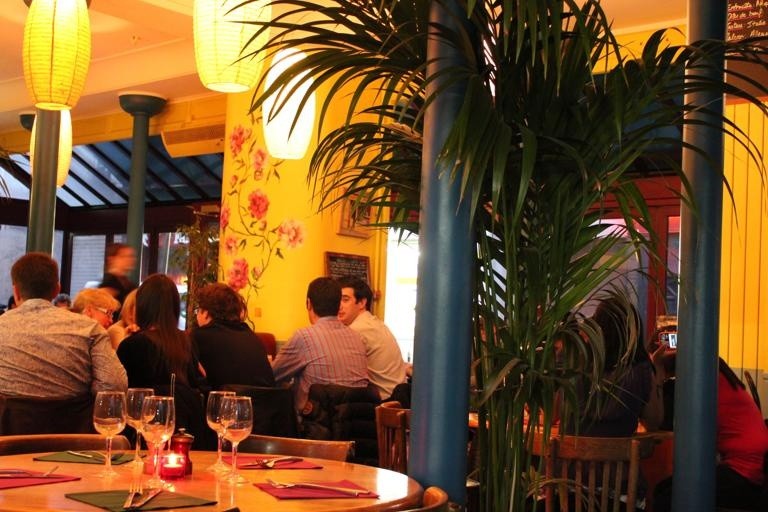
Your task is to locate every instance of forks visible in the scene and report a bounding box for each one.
[0,466,59,476]
[67,448,116,461]
[123,482,141,509]
[265,477,371,497]
[256,457,302,473]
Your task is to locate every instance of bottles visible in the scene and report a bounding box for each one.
[173,426,193,475]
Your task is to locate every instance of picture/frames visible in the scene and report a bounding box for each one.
[338,187,374,239]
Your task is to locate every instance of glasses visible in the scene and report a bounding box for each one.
[96,306,114,317]
[194,307,200,314]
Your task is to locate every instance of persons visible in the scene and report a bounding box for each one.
[53,294,72,308]
[117,272,192,449]
[473,297,674,437]
[269,278,368,417]
[337,274,406,400]
[75,288,122,328]
[7,296,16,309]
[190,282,277,451]
[649,355,767,509]
[99,244,140,352]
[0,252,128,400]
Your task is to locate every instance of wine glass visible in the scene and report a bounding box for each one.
[124,388,154,469]
[140,394,176,491]
[206,389,254,486]
[90,389,125,474]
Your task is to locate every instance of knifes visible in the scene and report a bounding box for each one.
[133,489,163,512]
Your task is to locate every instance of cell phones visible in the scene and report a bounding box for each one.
[658,332,678,350]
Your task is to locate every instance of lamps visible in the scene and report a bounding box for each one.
[22,0,92,111]
[30,110,72,187]
[192,0,273,93]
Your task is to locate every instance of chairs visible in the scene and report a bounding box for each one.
[222,434,355,462]
[528,433,654,512]
[397,486,449,512]
[222,382,295,436]
[0,433,131,456]
[375,401,481,512]
